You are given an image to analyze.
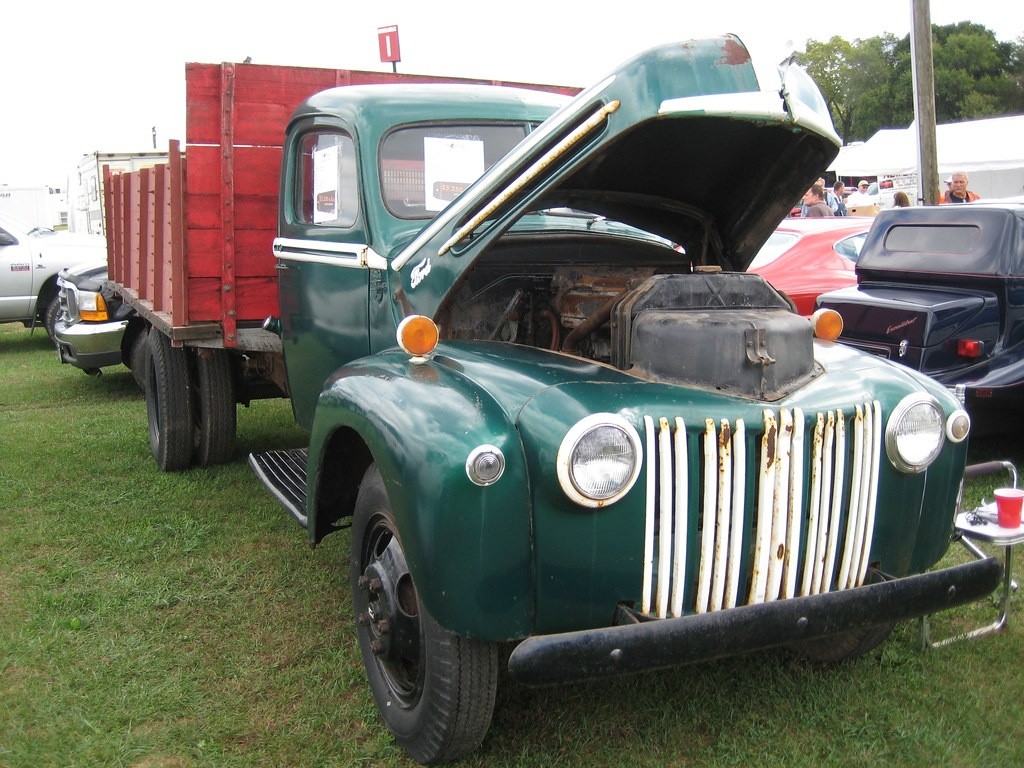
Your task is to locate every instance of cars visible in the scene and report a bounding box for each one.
[814,203,1024,445]
[54,259,148,390]
[0,224,108,343]
[673,216,875,316]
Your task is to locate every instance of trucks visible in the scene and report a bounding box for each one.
[102,31,1024,767]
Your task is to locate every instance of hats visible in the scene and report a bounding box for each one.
[858,180,870,186]
[944,175,952,183]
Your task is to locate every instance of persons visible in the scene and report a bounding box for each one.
[801,173,982,218]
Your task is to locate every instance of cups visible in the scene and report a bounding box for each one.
[994,488,1024,528]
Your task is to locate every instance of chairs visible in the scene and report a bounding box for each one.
[920,460,1024,648]
